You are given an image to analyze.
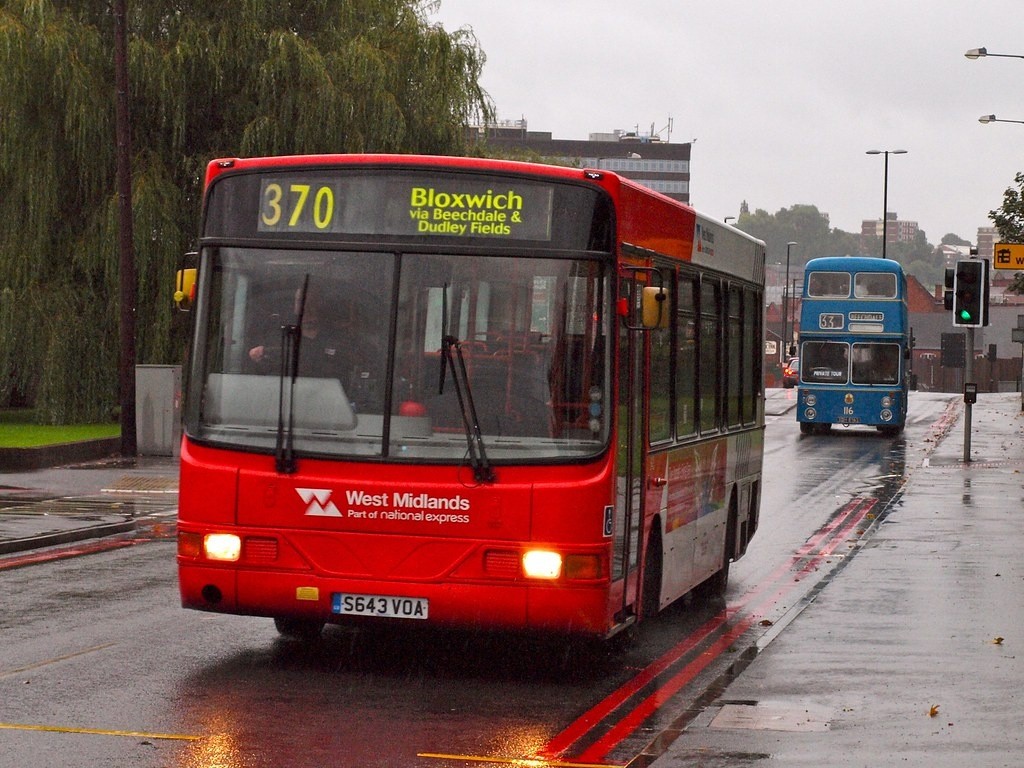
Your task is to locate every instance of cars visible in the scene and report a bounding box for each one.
[783,359,799,389]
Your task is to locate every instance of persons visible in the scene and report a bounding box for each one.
[250,286,354,392]
[809,346,837,373]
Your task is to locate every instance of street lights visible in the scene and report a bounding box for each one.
[865,149,908,259]
[783,241,798,362]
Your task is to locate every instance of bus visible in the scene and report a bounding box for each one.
[788,257,916,435]
[170,153,769,655]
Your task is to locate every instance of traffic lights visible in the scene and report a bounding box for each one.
[910,336,916,348]
[952,259,985,328]
[944,268,955,311]
